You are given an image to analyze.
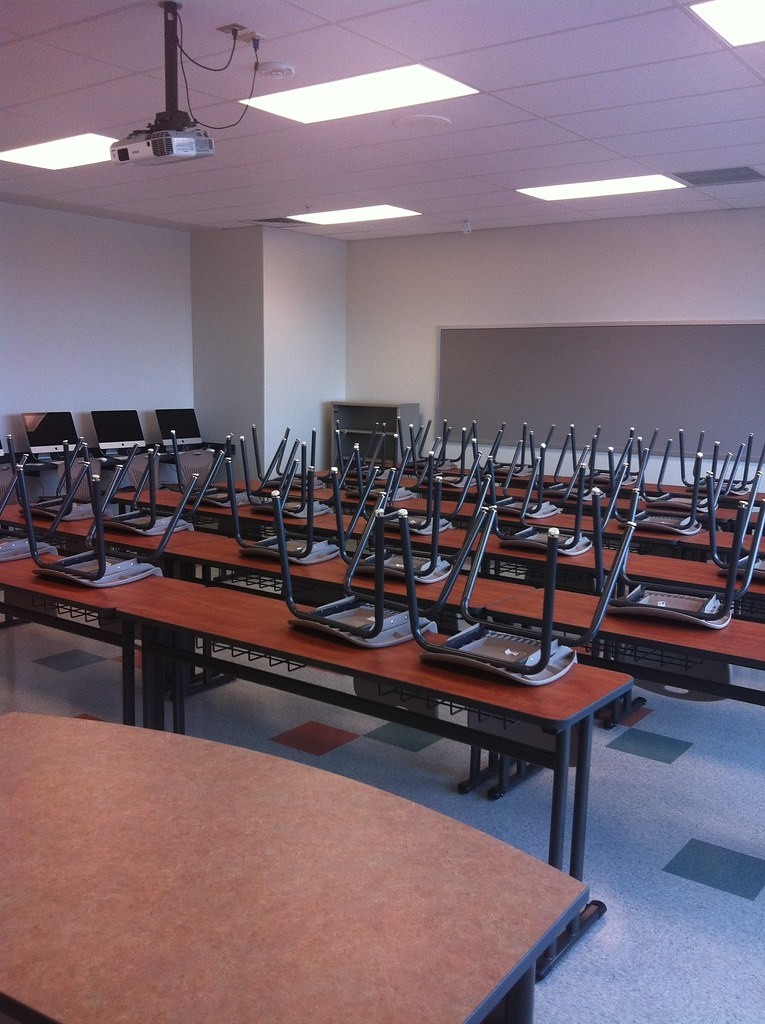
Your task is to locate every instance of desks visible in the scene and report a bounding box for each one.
[0,469,765,1023]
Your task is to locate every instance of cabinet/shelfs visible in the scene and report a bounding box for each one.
[330,400,421,471]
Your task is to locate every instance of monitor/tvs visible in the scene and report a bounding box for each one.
[21,412,81,464]
[155,408,202,455]
[91,410,146,460]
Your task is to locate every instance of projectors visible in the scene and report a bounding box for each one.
[110,128,214,167]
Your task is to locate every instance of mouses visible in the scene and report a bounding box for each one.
[208,448,215,453]
[148,449,154,451]
[98,457,107,462]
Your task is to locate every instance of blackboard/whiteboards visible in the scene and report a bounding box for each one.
[435,319,765,462]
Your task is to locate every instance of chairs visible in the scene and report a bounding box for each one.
[0,415,764,691]
